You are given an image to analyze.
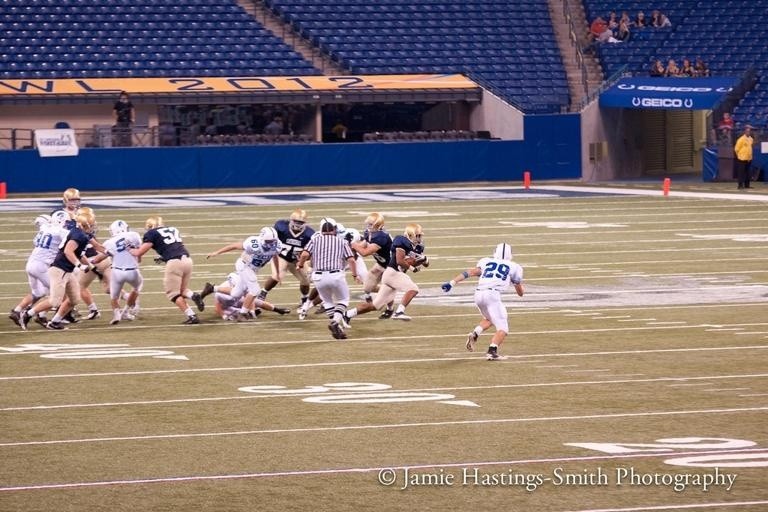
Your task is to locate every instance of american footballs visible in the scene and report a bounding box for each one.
[406,255,422,266]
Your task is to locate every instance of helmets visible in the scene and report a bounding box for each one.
[146,215,164,230]
[494,243,513,262]
[108,220,128,236]
[260,209,423,245]
[52,187,97,230]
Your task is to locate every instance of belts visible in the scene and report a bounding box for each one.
[477,288,497,290]
[315,269,339,274]
[114,267,135,271]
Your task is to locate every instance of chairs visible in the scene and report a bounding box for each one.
[0,1,767,144]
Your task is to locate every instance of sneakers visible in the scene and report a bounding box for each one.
[327,321,348,339]
[465,331,478,353]
[8,282,412,330]
[486,345,508,360]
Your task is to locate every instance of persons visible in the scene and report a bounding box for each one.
[112,90,136,146]
[718,111,735,144]
[189,109,348,144]
[652,56,711,77]
[734,128,755,190]
[440,241,524,360]
[590,9,671,42]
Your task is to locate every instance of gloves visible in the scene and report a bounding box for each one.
[92,266,103,278]
[76,263,90,273]
[442,280,457,293]
[409,264,421,273]
[154,256,164,264]
